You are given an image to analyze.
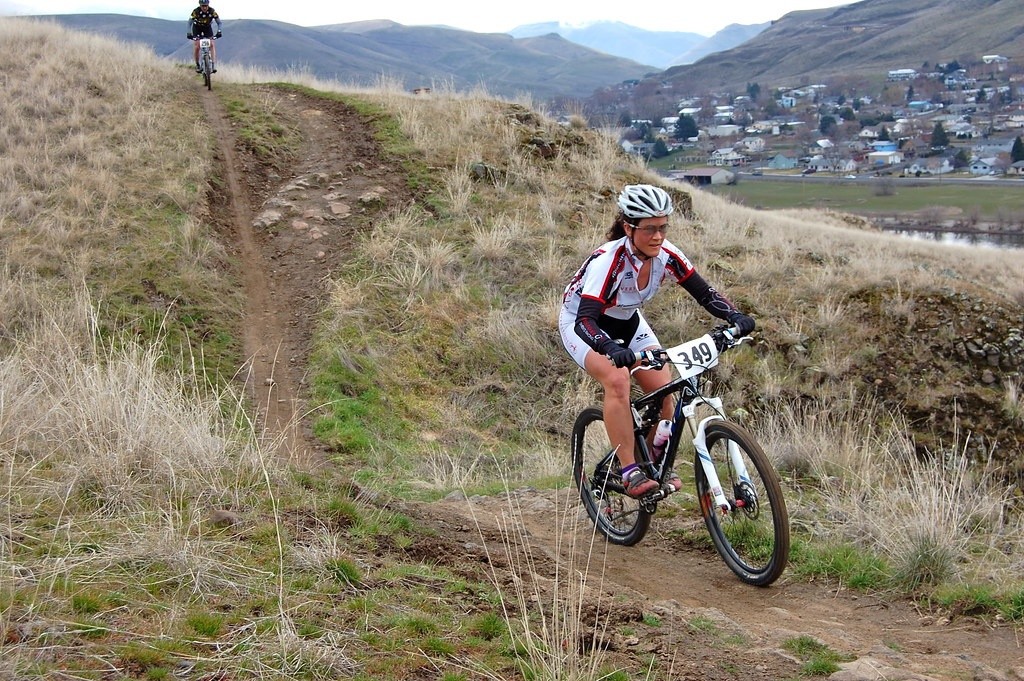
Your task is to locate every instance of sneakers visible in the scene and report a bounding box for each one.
[649,449,682,490]
[622,467,659,496]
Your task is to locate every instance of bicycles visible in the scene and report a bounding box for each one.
[186,33,221,91]
[569,320,793,588]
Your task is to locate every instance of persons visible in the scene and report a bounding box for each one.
[186,0,222,73]
[559,185,755,499]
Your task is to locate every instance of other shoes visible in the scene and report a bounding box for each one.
[195,65,200,71]
[211,69,217,73]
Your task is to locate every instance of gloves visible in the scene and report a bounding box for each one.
[727,312,755,337]
[602,343,637,368]
[186,32,195,40]
[212,33,222,39]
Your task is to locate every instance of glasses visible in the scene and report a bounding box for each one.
[202,5,208,7]
[623,220,671,235]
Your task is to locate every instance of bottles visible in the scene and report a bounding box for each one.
[649,419,673,464]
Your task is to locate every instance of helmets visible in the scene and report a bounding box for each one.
[617,184,673,218]
[199,0,209,5]
